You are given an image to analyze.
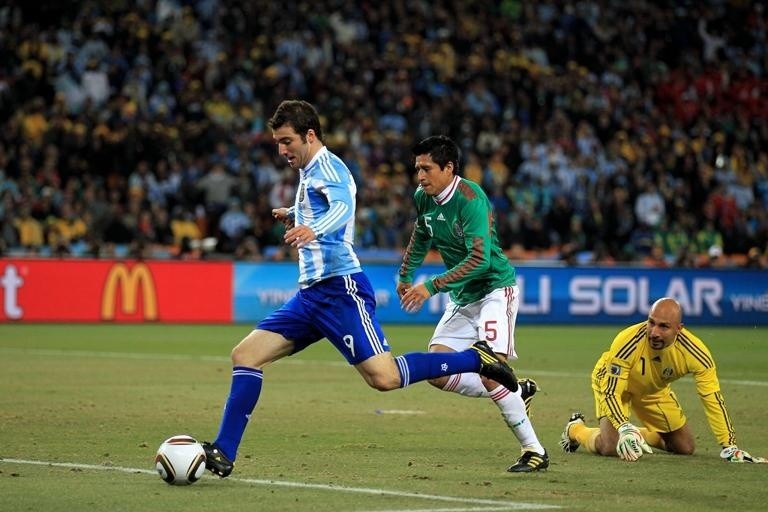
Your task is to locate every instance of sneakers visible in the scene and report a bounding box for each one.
[200,440,233,477]
[469,341,585,472]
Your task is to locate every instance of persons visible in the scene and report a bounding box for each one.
[198,98,521,482]
[559,295,768,464]
[395,133,549,472]
[2,2,768,269]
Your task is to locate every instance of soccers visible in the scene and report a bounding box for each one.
[155,434,207,486]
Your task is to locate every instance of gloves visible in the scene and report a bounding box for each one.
[616,424,653,461]
[719,446,768,464]
